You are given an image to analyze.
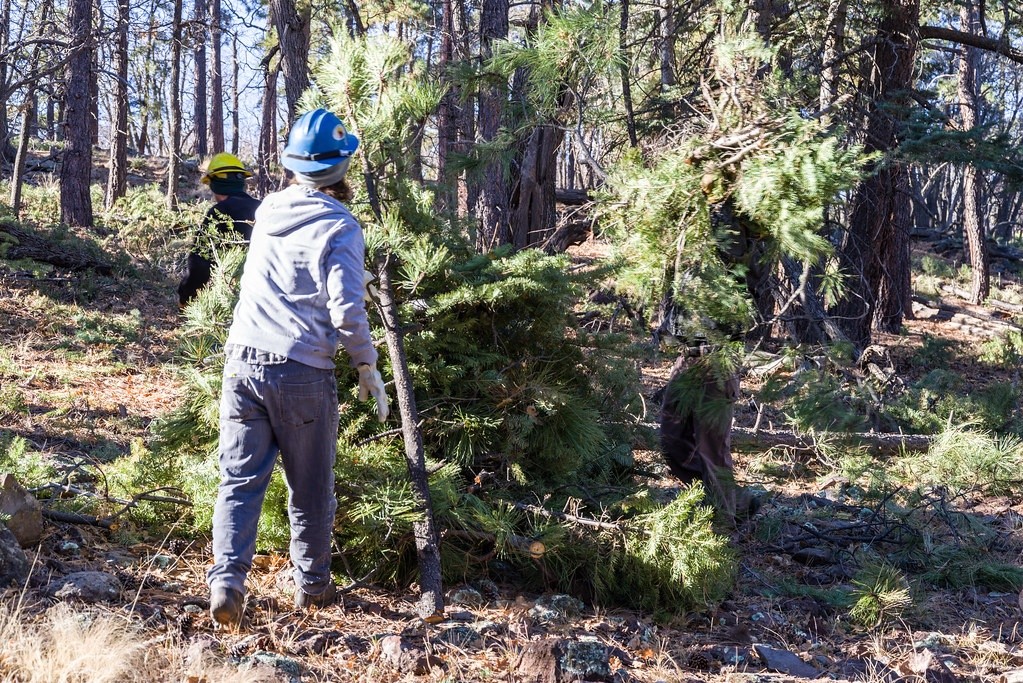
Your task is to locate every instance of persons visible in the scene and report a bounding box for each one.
[209,107,389,622]
[178,153,262,304]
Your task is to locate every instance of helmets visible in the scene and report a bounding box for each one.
[202,152,252,184]
[281,109,359,174]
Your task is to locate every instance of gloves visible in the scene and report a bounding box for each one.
[356,365,389,422]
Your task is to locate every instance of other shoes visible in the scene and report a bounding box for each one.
[294,581,339,611]
[208,588,248,625]
